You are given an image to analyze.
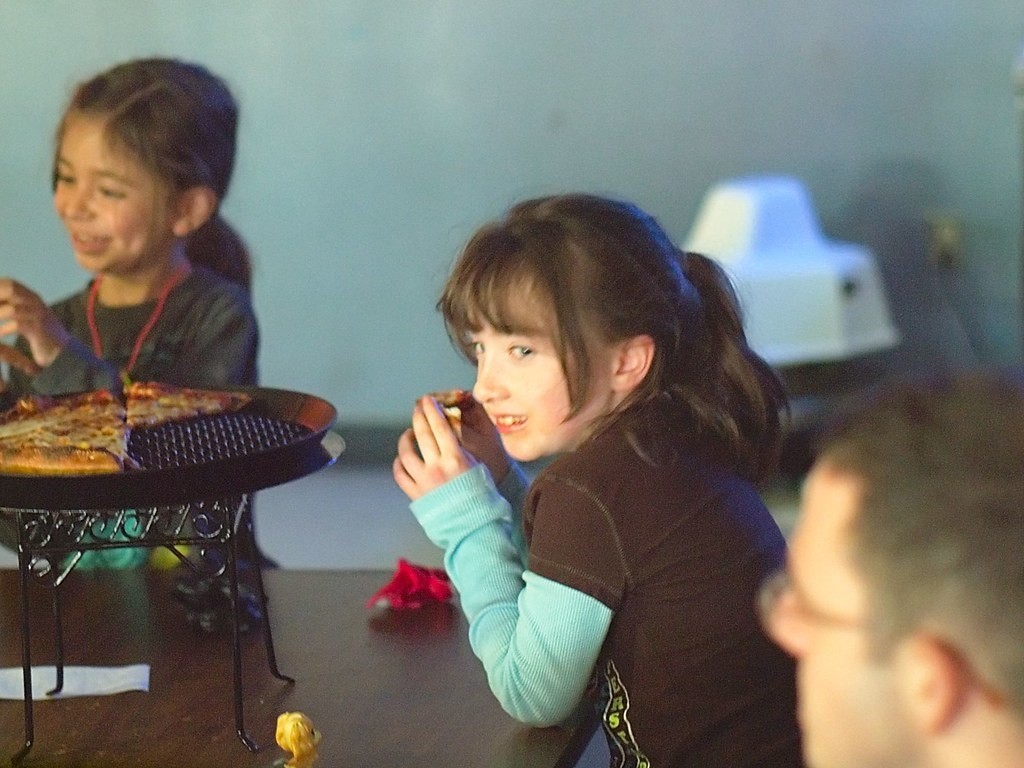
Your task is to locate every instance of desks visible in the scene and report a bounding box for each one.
[0,567,599,768]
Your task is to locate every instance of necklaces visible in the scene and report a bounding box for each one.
[88,265,188,372]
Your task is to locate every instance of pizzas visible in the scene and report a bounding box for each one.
[0,386,130,476]
[413,389,472,411]
[124,381,251,428]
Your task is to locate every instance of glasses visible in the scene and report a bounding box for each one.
[751,564,876,635]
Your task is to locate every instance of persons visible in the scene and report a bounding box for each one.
[788,383,1024,768]
[393,194,803,767]
[0,57,272,571]
